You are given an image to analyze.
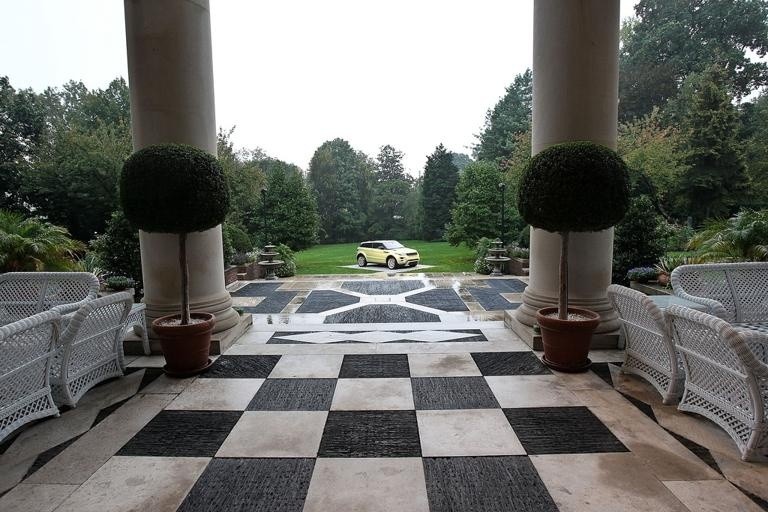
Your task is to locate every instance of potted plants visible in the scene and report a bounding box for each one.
[118,140,236,378]
[514,140,635,371]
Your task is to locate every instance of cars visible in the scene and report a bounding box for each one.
[356,239,419,270]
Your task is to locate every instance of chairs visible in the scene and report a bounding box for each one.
[602,260,767,465]
[0,267,136,441]
